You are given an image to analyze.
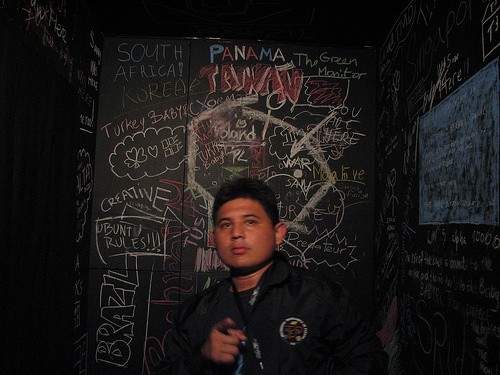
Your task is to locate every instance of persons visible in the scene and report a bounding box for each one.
[167,177,373,375]
